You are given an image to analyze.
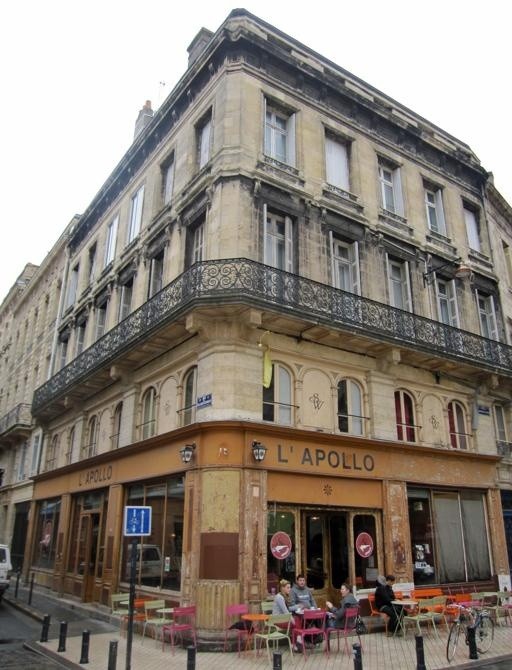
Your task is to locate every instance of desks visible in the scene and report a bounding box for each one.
[241,614,268,655]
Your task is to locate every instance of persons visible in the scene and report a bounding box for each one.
[41,519,53,540]
[289,573,323,648]
[373,575,408,637]
[271,576,303,654]
[317,583,358,652]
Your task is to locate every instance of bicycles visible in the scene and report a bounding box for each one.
[446,604,495,663]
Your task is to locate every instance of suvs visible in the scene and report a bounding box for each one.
[1,544,13,598]
[79,543,164,583]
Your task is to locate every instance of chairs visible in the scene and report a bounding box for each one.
[261,602,365,662]
[368,589,512,639]
[109,593,183,651]
[162,606,197,657]
[254,613,294,668]
[224,605,259,658]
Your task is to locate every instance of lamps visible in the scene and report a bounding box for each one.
[421,255,473,289]
[252,440,267,461]
[180,442,197,464]
